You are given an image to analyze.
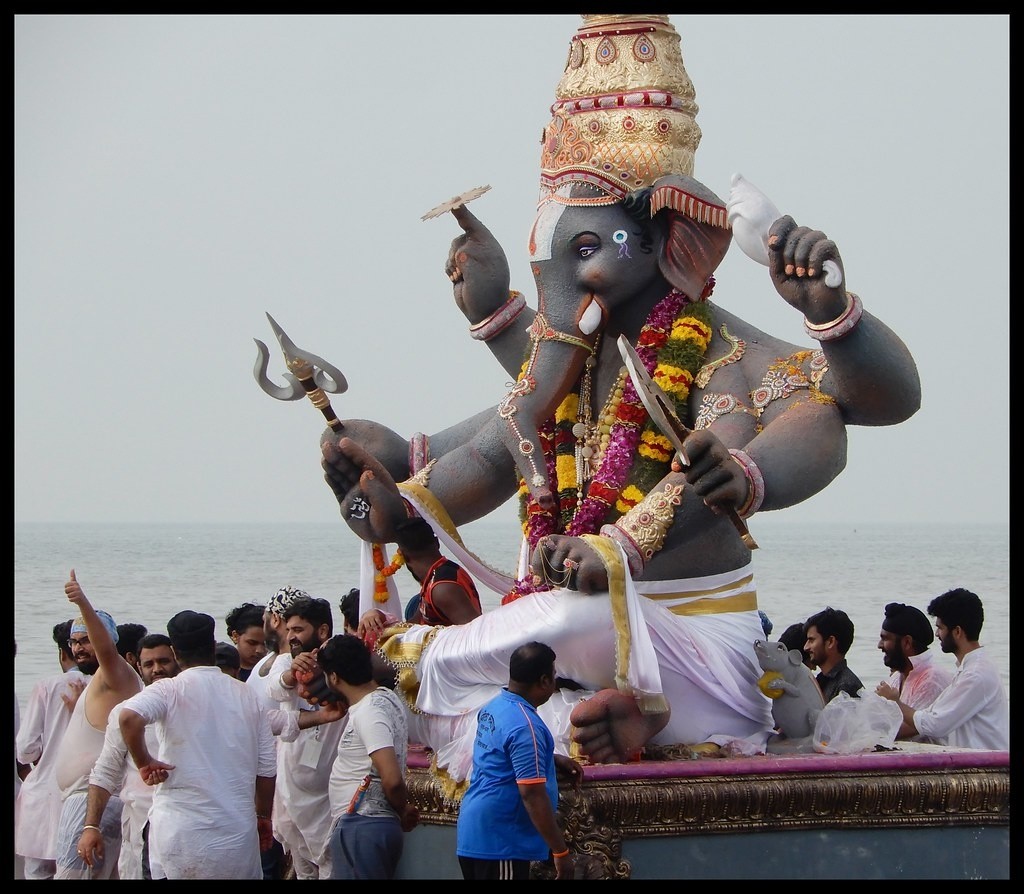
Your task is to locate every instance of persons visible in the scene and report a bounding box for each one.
[14,527,581,880]
[760,589,1010,754]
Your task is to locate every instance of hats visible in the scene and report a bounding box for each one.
[212,643,241,669]
[266,586,311,621]
[71,609,119,645]
[881,603,934,646]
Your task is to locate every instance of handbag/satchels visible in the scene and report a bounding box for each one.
[813,688,903,755]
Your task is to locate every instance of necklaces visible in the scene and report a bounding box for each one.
[511,272,716,605]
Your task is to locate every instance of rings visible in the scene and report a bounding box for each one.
[78,850,83,853]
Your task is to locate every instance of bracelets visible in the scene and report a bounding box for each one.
[728,449,764,519]
[599,521,645,580]
[471,289,527,341]
[803,292,863,343]
[83,824,101,834]
[281,670,295,688]
[256,813,272,821]
[552,848,570,859]
[371,498,420,602]
[407,434,432,476]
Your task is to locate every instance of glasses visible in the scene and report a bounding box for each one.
[67,636,90,648]
